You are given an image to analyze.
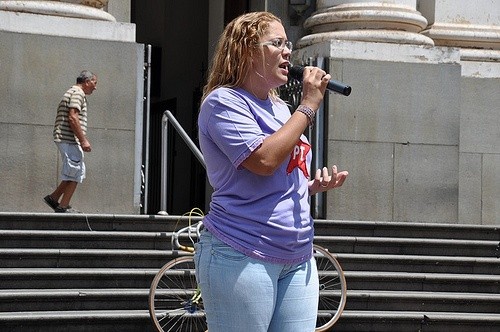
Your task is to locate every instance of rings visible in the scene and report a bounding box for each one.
[320,183,329,188]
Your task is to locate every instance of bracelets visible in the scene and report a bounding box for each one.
[297,105,316,130]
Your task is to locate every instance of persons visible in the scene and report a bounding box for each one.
[43,70,98,213]
[193,11,349,332]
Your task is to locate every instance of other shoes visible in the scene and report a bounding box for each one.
[55,205,84,213]
[42,195,61,211]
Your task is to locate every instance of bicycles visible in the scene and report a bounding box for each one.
[150,208,347,332]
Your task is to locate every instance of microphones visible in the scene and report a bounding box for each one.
[290,65,351,96]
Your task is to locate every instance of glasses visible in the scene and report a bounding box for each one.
[254,38,293,52]
